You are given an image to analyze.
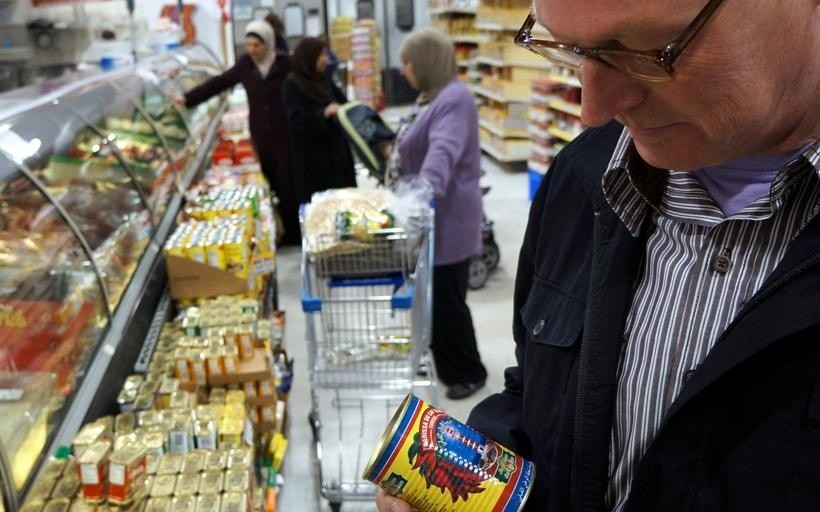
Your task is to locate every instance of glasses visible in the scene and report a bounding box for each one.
[513,2,726,83]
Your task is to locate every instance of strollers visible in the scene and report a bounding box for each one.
[329,98,501,291]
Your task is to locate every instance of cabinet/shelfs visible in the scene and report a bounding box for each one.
[430,0,591,180]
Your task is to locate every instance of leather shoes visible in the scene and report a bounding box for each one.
[447,380,484,398]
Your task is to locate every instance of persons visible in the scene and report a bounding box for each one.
[376,0,820,509]
[386,28,489,400]
[82,31,133,68]
[166,13,359,245]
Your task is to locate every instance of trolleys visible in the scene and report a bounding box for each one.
[298,182,437,512]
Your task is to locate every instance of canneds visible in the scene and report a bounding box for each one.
[43,184,285,512]
[362,392,538,512]
